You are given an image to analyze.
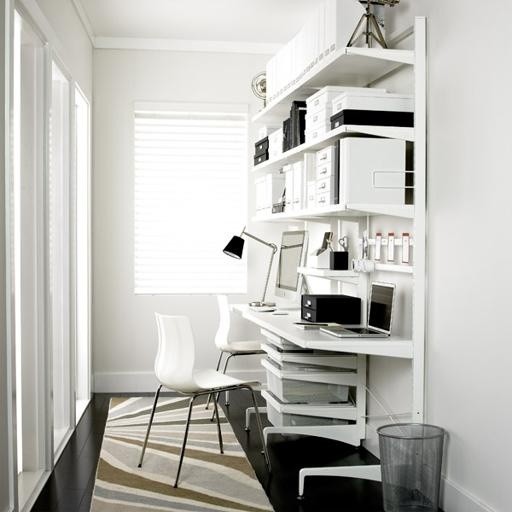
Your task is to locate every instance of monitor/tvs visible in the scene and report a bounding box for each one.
[274,230,310,302]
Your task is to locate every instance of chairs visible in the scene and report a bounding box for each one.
[138,297,261,488]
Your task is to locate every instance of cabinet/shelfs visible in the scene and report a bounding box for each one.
[244,14,425,226]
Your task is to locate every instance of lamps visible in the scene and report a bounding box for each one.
[222,225,280,316]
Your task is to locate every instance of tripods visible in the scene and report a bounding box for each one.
[346,0,389,49]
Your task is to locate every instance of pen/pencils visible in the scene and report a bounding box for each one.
[272,314,288,315]
[295,323,328,326]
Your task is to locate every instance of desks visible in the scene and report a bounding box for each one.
[227,296,423,500]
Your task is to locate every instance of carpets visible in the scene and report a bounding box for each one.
[93,392,274,512]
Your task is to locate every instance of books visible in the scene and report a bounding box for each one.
[282,100,307,153]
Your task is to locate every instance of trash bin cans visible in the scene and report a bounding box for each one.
[377,423,445,512]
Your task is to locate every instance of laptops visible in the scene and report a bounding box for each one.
[321,281,396,338]
